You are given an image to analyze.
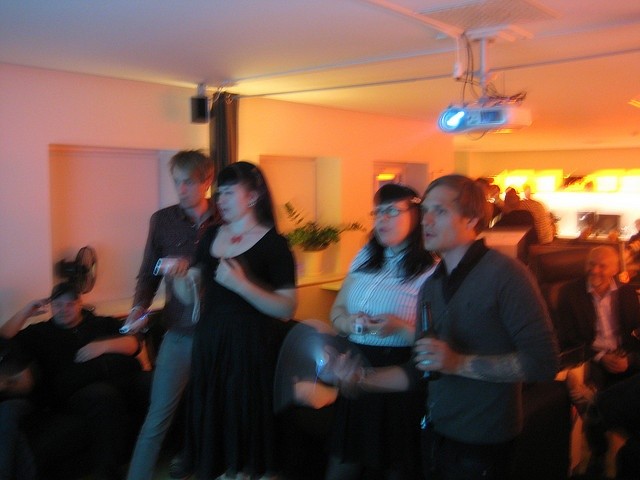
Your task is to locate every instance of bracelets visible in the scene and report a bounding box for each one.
[130,340,143,358]
[333,314,350,338]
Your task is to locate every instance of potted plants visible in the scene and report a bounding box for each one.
[284,202,367,275]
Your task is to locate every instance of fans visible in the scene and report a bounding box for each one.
[58,246,97,313]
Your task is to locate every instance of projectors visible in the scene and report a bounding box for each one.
[436,23,533,136]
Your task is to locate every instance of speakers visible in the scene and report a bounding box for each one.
[190,96,208,124]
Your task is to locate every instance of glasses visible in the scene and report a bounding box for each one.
[367,208,401,221]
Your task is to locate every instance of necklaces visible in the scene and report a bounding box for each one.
[223,222,263,244]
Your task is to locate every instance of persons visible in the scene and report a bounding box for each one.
[335,175,559,480]
[629,218,640,263]
[330,182,438,480]
[125,150,225,480]
[475,178,554,245]
[167,160,297,480]
[560,244,640,480]
[0,281,152,480]
